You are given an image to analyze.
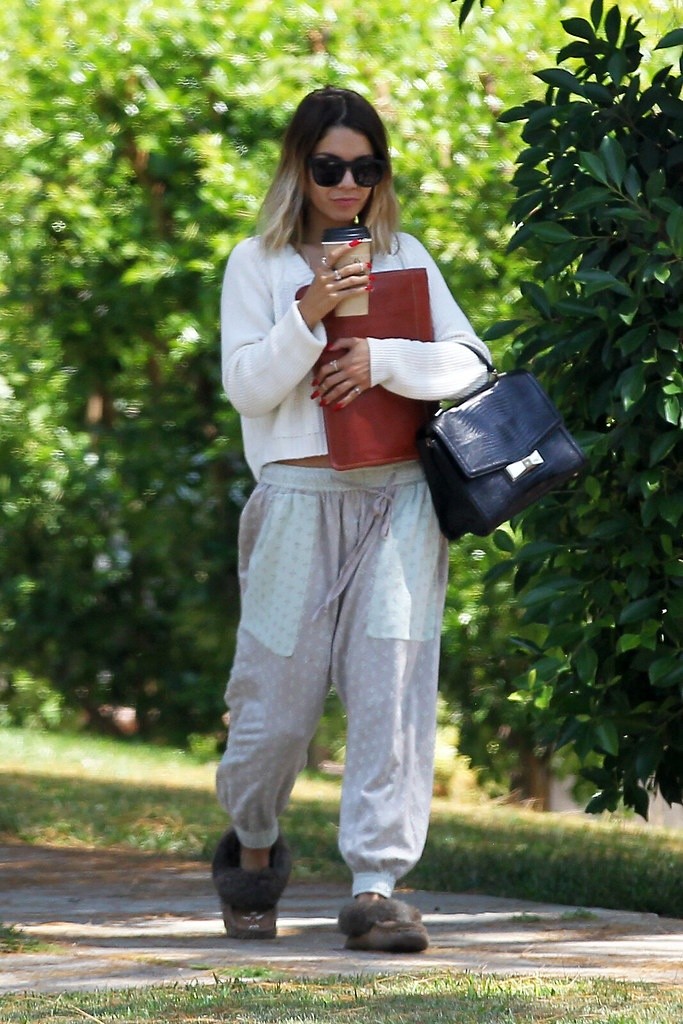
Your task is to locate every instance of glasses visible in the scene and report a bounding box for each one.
[307,156,385,187]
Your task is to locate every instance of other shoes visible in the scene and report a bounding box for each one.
[336,899,430,955]
[211,828,293,939]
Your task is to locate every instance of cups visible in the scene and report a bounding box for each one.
[321,227,373,316]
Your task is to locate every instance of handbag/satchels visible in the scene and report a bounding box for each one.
[415,343,587,541]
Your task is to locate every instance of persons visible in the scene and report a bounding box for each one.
[211,81,496,952]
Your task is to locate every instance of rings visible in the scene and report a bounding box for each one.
[330,360,338,371]
[359,262,365,274]
[334,269,342,281]
[320,256,333,268]
[353,387,361,395]
